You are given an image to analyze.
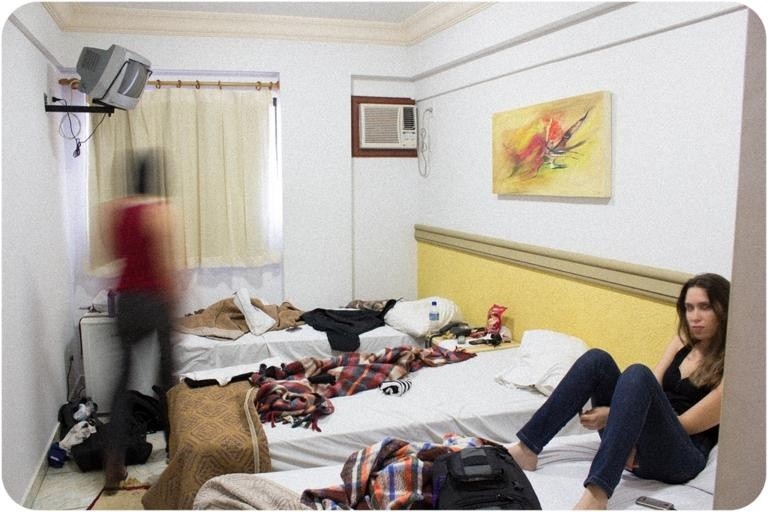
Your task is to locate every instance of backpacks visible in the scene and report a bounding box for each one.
[434,446,543,509]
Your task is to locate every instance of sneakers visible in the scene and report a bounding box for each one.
[104,467,128,495]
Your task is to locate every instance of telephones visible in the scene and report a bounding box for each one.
[441,322,472,336]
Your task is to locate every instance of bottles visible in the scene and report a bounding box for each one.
[428,300,441,334]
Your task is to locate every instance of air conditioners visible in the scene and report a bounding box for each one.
[357,101,419,153]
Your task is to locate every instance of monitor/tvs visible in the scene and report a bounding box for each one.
[76,43,152,111]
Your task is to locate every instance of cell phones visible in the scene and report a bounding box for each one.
[635,495,673,510]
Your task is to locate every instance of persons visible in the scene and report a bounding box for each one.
[103,149,187,491]
[504,273,731,510]
